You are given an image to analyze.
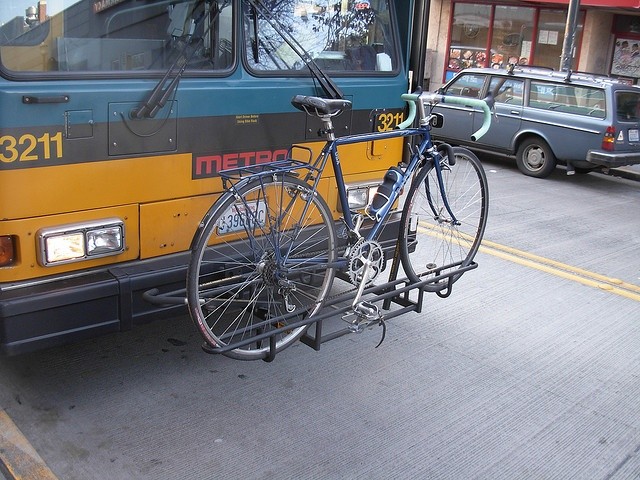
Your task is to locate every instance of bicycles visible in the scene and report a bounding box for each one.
[185,93,491,361]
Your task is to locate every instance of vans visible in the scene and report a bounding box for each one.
[416,67,640,178]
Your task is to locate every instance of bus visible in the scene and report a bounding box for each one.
[2,1,416,365]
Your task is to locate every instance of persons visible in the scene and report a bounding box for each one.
[631,43,639,56]
[622,40,628,50]
